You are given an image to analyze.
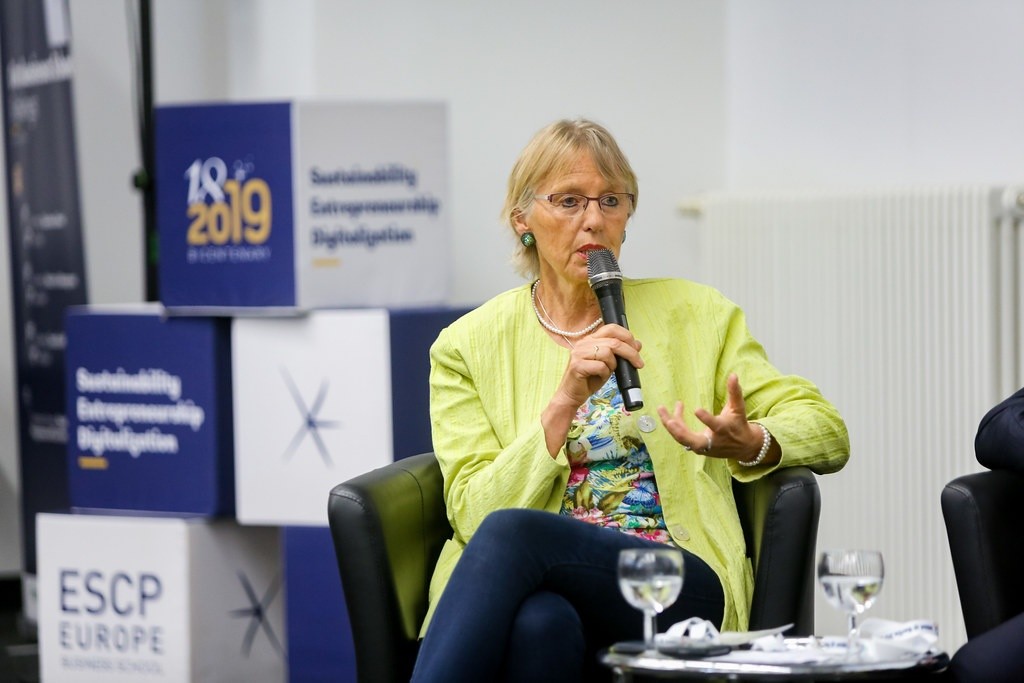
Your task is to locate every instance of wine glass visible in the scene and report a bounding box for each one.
[818,549,885,636]
[619,548,686,645]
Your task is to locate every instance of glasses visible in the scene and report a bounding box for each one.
[533,193,634,219]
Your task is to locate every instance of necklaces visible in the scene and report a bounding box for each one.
[536,291,599,349]
[532,280,603,338]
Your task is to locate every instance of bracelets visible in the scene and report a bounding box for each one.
[737,424,770,466]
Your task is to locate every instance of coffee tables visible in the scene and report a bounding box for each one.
[601,635,949,683]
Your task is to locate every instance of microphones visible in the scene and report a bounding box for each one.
[588,248,644,412]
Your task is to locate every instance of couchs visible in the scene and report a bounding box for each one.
[327,453,822,683]
[940,471,1023,639]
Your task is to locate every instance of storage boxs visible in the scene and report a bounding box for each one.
[227,302,467,530]
[34,512,286,683]
[65,303,235,522]
[283,526,354,683]
[152,97,450,318]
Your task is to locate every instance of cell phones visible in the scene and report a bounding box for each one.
[610,641,731,659]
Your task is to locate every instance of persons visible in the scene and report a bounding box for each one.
[413,118,850,683]
[944,387,1024,683]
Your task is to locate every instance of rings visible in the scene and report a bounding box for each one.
[686,446,690,451]
[593,346,598,360]
[704,435,712,452]
